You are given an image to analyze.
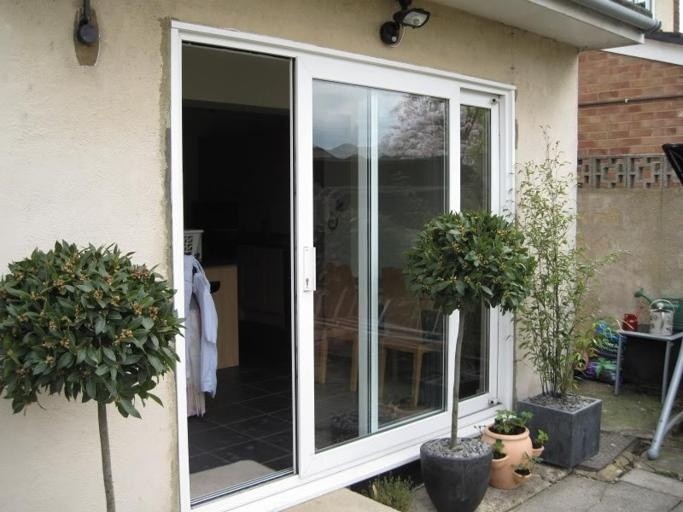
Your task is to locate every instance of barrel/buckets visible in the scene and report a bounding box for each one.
[647,297,675,336]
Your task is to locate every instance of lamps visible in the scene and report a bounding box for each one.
[375,1,433,49]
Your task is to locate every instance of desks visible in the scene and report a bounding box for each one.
[610,317,682,405]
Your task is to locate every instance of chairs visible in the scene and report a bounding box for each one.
[302,264,445,410]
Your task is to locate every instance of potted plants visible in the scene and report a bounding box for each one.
[471,405,551,491]
[395,207,547,511]
[486,117,637,471]
